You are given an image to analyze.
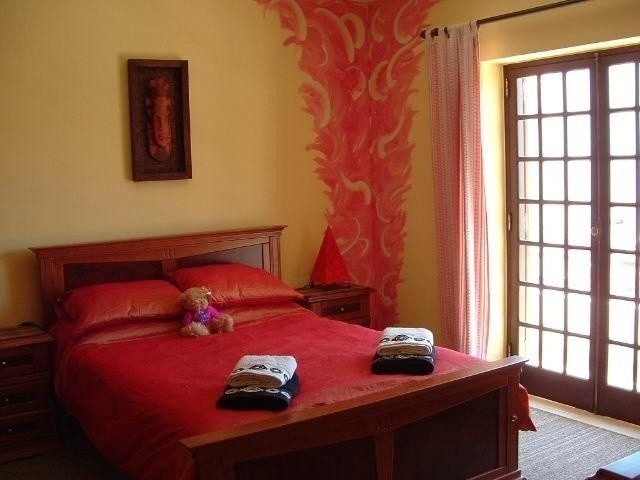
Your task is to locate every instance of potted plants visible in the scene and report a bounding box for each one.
[127,57,193,181]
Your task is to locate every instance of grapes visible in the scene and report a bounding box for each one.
[0,326,64,465]
[292,283,376,329]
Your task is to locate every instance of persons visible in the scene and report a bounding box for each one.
[152,109,173,149]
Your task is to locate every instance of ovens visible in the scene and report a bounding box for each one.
[28,224,531,479]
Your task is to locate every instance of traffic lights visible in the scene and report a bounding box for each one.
[52,262,306,339]
